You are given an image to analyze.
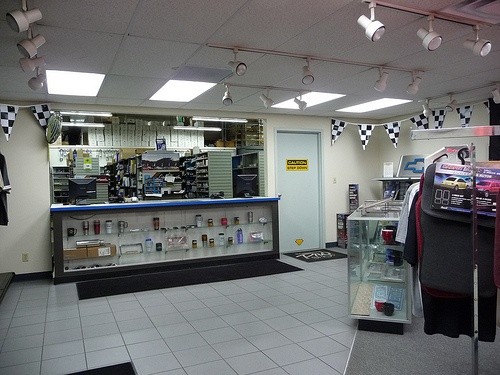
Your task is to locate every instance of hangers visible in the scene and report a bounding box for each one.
[457,148,470,164]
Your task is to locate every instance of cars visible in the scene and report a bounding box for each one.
[440,176,469,191]
[465,176,480,190]
[475,176,500,198]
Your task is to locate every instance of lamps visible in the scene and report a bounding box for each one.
[59,111,113,127]
[173,0,500,131]
[7,0,47,89]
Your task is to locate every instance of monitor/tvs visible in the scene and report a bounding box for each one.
[68,178,97,200]
[235,175,260,198]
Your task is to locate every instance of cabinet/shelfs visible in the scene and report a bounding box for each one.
[346,200,413,336]
[372,177,421,199]
[51,145,280,285]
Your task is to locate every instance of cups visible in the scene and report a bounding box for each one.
[118,221,128,233]
[106,220,112,233]
[382,226,403,266]
[195,215,202,227]
[83,220,89,236]
[146,239,153,252]
[94,220,100,234]
[221,218,227,225]
[208,219,213,226]
[156,243,162,251]
[67,228,77,237]
[248,212,253,223]
[234,217,239,224]
[375,299,395,316]
[153,217,159,230]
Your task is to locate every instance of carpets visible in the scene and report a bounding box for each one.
[69,362,138,375]
[344,315,500,375]
[76,258,304,300]
[284,249,347,263]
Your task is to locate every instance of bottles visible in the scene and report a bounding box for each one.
[237,228,243,244]
[192,233,234,248]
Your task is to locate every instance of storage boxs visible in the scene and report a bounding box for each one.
[87,245,116,258]
[86,117,205,149]
[64,248,87,259]
